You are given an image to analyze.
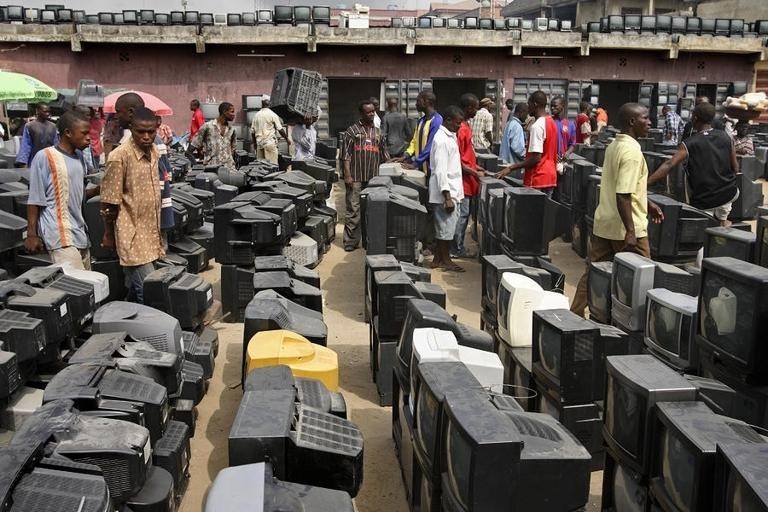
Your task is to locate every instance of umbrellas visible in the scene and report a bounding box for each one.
[0,71,58,140]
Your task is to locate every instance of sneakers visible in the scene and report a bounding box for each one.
[345,245,355,251]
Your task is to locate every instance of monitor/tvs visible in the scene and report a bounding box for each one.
[0,266,219,511]
[357,81,768,263]
[0,82,339,325]
[363,254,594,512]
[392,14,768,35]
[198,288,361,512]
[0,268,109,412]
[0,5,333,25]
[481,253,768,512]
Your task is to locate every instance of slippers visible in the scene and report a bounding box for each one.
[431,256,465,272]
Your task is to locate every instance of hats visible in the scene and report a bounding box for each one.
[480,98,492,105]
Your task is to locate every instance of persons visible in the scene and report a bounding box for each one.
[341,98,413,251]
[570,102,664,319]
[647,97,753,222]
[390,90,607,270]
[184,100,322,173]
[16,92,173,302]
